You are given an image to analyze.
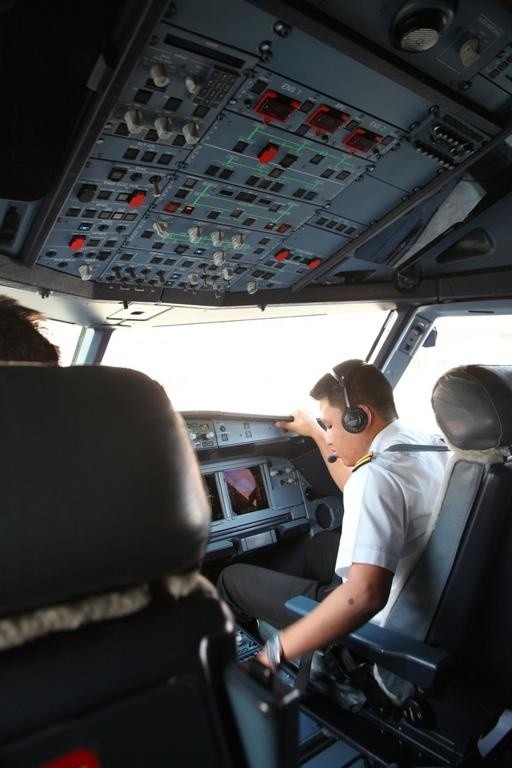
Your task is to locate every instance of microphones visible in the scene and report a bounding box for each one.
[327,453,341,467]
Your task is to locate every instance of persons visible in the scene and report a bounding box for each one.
[257,358,453,676]
[0,295,61,362]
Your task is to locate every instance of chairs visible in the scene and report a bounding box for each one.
[0,361,301,768]
[257,364,512,768]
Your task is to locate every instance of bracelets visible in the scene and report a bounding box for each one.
[264,635,282,672]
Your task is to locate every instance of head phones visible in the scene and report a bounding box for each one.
[327,367,368,435]
